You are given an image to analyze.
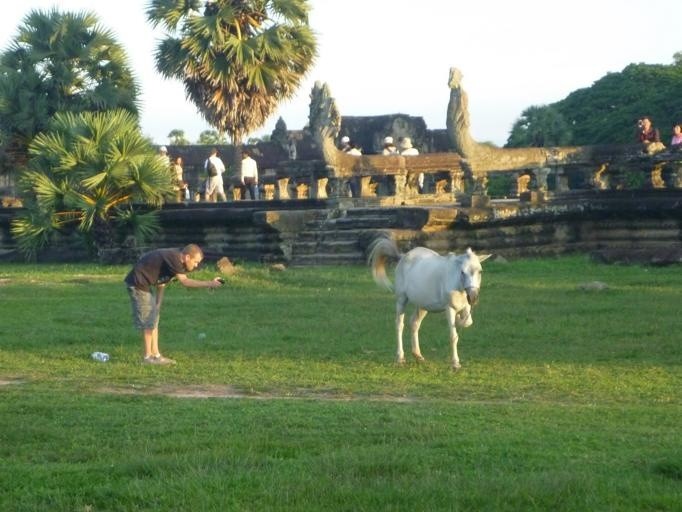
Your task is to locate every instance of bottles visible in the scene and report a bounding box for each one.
[90,352,109,362]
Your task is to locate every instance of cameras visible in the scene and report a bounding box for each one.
[217,278,225,284]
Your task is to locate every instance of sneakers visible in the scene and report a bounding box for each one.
[157,355,177,365]
[143,354,169,365]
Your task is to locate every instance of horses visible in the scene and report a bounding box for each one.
[366,233,494,373]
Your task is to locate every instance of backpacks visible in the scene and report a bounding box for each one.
[208,158,217,176]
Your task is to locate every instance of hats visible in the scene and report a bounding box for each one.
[159,146,168,153]
[341,136,349,143]
[400,138,413,148]
[384,136,393,144]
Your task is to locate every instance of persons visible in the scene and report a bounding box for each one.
[201,147,228,202]
[381,136,400,156]
[634,115,660,144]
[667,122,681,147]
[124,242,223,367]
[239,149,261,200]
[170,157,185,201]
[155,146,173,175]
[338,135,353,153]
[400,138,419,157]
